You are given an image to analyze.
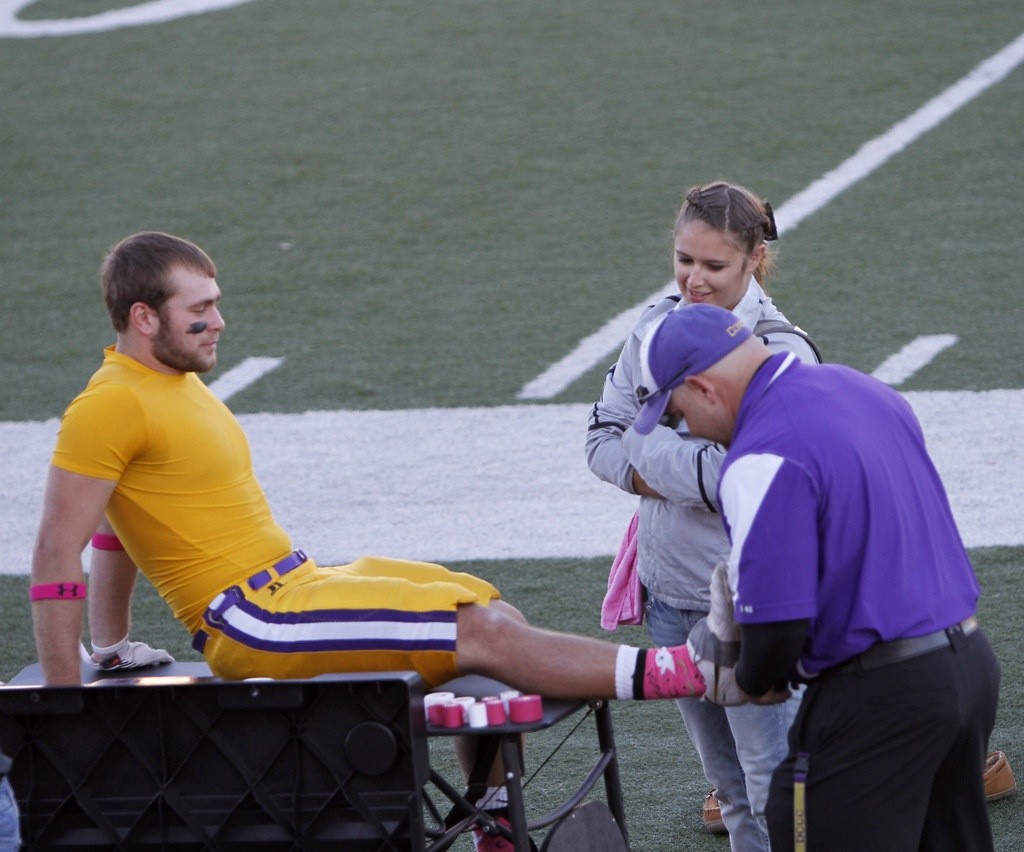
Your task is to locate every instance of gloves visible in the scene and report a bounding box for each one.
[91,633,175,670]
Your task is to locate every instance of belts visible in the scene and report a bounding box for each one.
[816,615,978,678]
[192,549,307,653]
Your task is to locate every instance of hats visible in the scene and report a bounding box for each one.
[633,302,751,436]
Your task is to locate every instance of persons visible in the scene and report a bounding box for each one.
[632,302,1000,852]
[585,182,822,852]
[30,231,741,851]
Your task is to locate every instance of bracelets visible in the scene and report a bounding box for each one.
[89,532,128,551]
[29,581,87,601]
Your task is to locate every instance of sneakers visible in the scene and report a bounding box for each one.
[703,785,727,834]
[686,561,752,706]
[472,816,538,852]
[983,750,1015,803]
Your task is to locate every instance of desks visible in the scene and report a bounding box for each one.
[4,658,631,852]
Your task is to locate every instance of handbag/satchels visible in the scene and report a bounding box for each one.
[601,510,647,631]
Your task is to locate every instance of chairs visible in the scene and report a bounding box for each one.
[0,677,426,852]
